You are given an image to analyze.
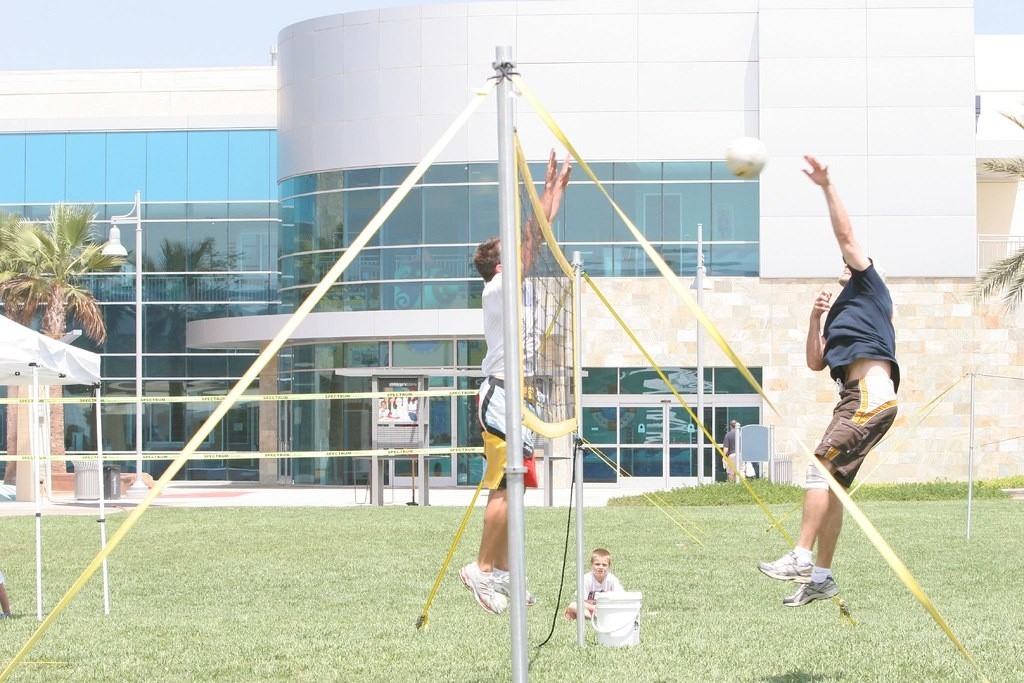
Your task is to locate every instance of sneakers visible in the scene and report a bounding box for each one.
[459,562,507,615]
[494,568,536,606]
[758,550,814,584]
[783,575,839,607]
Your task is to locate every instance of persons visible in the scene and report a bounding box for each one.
[758,155,900,608]
[379,396,418,420]
[722,421,741,480]
[459,148,572,615]
[0,572,11,617]
[566,549,624,620]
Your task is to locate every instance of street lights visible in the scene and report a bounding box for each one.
[102,190,151,497]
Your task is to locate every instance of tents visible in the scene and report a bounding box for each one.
[0,315,110,621]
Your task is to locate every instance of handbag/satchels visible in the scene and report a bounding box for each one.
[745,462,755,477]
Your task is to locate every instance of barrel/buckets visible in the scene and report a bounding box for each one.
[591,591,643,648]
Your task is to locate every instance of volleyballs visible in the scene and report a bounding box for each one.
[725,142,767,180]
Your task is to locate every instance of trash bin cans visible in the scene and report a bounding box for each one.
[104,464,121,500]
[71,460,100,500]
